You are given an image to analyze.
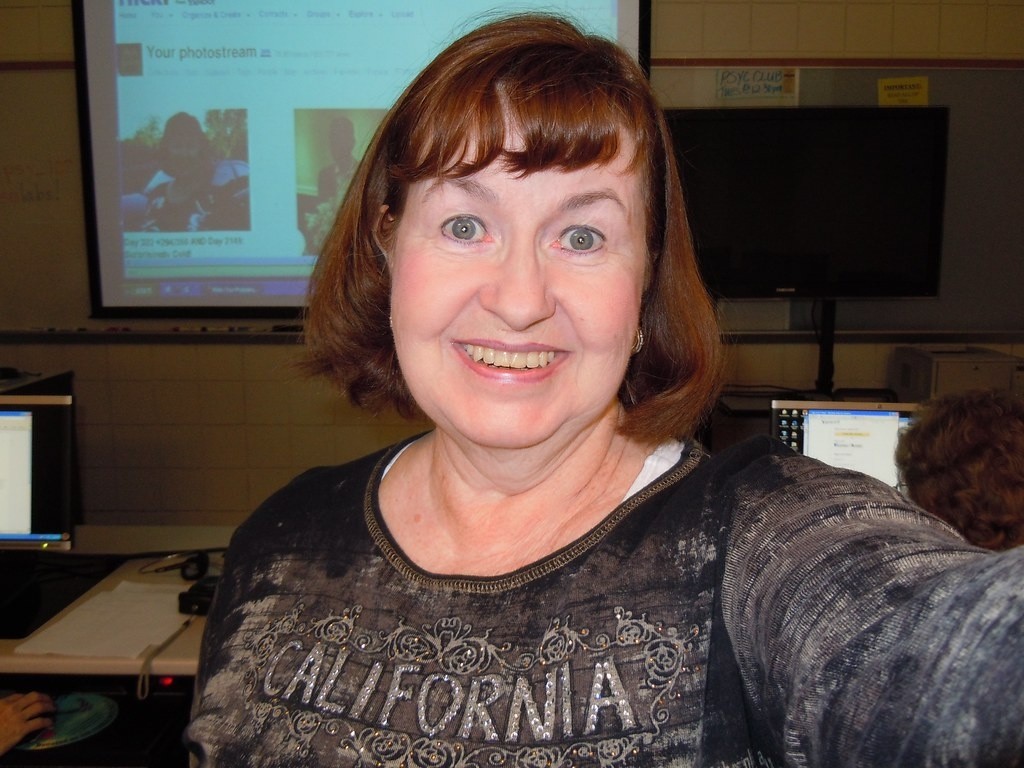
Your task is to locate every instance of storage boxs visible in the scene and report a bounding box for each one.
[898,344,1021,397]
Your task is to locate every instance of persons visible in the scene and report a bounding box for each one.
[184,15,1024,768]
[318,113,363,196]
[0,689,57,756]
[122,108,250,232]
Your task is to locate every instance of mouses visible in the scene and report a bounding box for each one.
[20,711,52,744]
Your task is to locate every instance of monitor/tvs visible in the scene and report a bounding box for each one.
[0,395,76,549]
[770,400,925,488]
[664,106,951,301]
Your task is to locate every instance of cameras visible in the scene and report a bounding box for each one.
[178,576,219,615]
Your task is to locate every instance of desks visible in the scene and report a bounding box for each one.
[0,524,240,676]
